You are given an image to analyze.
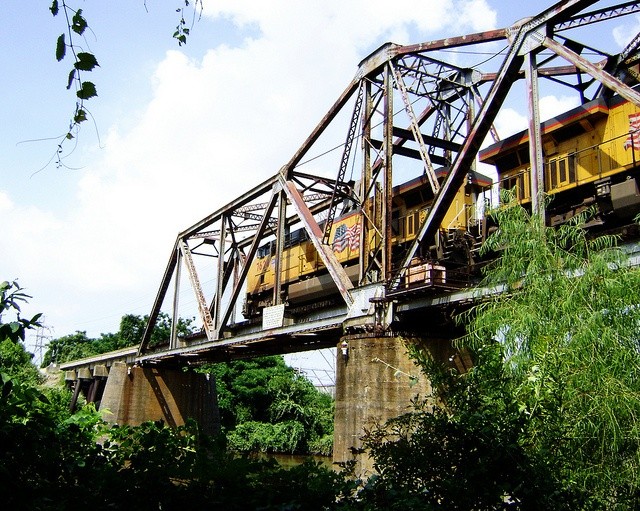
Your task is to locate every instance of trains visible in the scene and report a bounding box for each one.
[246,85,640,319]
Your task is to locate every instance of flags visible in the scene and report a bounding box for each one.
[623,112,640,150]
[332,223,361,251]
